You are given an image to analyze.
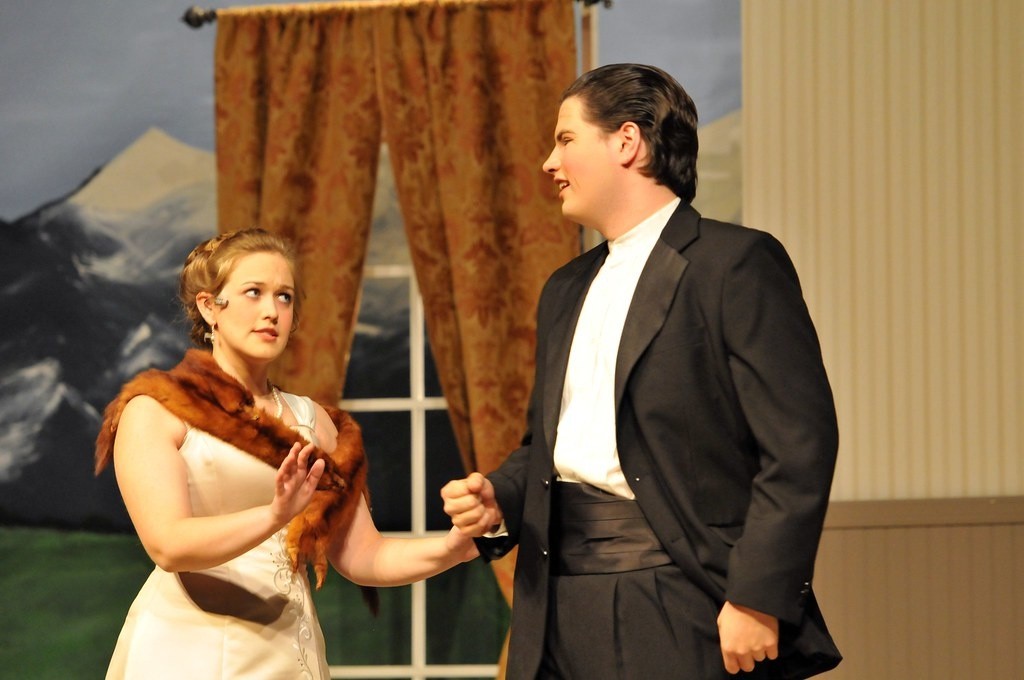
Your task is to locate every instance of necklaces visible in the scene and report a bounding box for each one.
[266,377,283,420]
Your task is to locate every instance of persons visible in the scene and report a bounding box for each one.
[442,64,844,680]
[94,227,480,680]
